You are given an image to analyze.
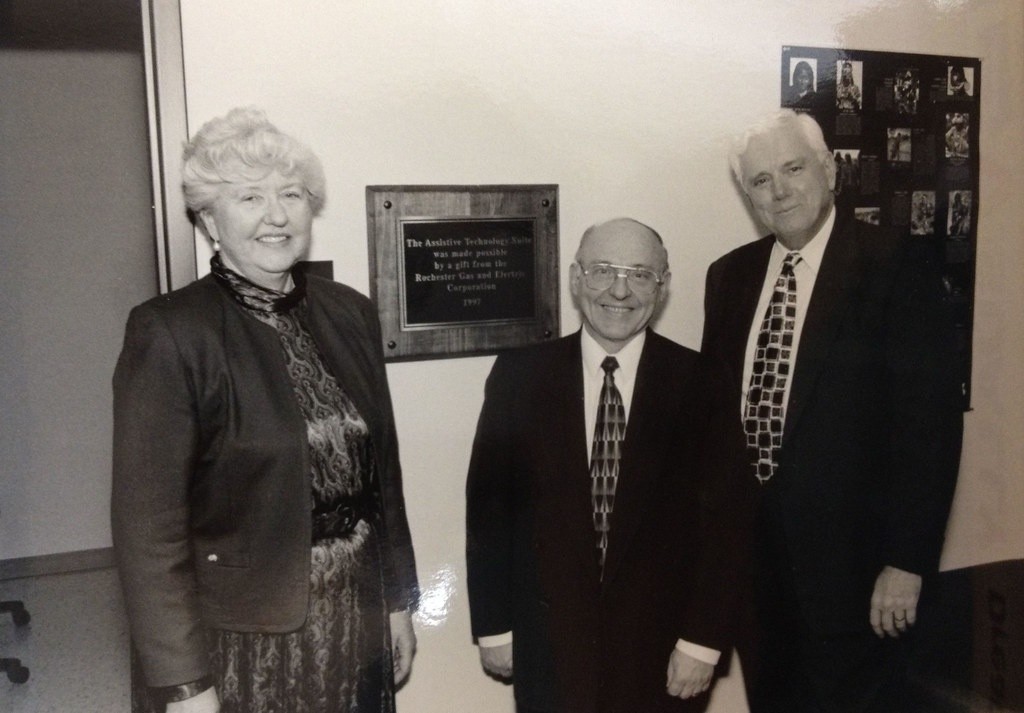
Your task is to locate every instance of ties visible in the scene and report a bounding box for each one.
[743,252,802,484]
[590,357,626,585]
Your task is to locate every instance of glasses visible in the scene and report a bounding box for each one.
[578,261,662,295]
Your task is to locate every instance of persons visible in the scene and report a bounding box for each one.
[789,61,816,109]
[109,104,420,713]
[832,114,970,238]
[836,62,860,112]
[949,66,970,96]
[464,217,759,713]
[701,108,964,713]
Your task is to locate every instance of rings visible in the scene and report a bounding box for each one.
[895,617,905,621]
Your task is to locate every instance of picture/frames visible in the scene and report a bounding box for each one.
[366,185,560,365]
